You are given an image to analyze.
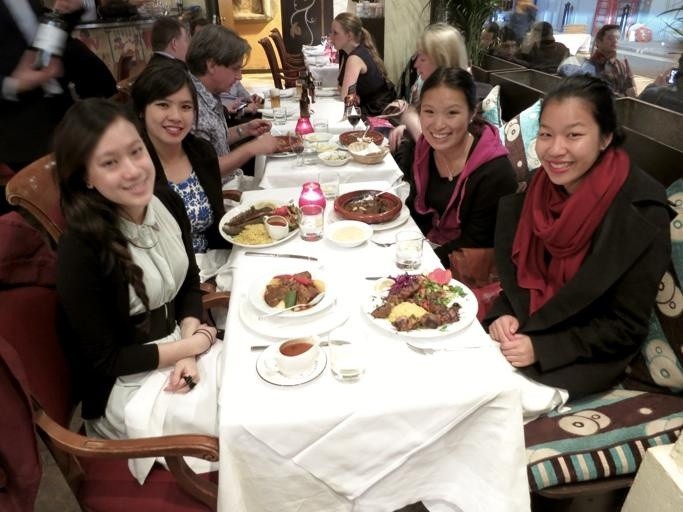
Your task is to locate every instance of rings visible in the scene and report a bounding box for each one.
[182,376,192,383]
[188,382,196,387]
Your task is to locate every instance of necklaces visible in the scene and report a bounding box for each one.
[438,134,473,182]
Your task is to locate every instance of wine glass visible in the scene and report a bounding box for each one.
[347,106,362,130]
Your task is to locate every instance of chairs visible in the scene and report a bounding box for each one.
[0,210,231,512]
[269,32,305,83]
[5,153,225,340]
[258,37,306,90]
[558,55,587,75]
[116,75,137,95]
[271,28,303,58]
[394,53,420,102]
[524,178,682,512]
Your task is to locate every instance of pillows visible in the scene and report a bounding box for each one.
[476,84,505,148]
[499,97,544,182]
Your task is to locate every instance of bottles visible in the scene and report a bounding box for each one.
[31,1,84,71]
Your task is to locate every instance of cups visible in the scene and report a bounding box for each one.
[393,230,423,269]
[273,108,287,125]
[263,214,289,240]
[327,327,368,380]
[318,170,340,198]
[313,118,329,133]
[277,335,318,372]
[315,52,324,67]
[298,182,326,242]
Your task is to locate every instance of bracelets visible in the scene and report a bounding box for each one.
[192,328,214,343]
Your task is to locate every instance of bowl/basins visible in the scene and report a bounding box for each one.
[310,141,340,152]
[339,130,385,145]
[348,141,391,164]
[319,151,352,165]
[326,221,373,248]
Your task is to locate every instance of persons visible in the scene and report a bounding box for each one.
[485,73,673,402]
[479,1,683,112]
[331,12,397,122]
[403,68,518,271]
[1,0,283,216]
[55,98,219,484]
[388,22,467,173]
[133,63,232,325]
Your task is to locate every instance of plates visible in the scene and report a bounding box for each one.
[328,203,412,232]
[315,89,340,97]
[305,132,332,142]
[245,262,336,316]
[362,271,480,343]
[220,198,301,248]
[264,134,306,158]
[334,189,403,221]
[263,108,294,118]
[263,89,294,98]
[256,341,327,385]
